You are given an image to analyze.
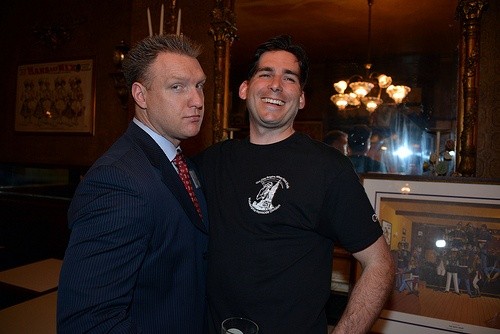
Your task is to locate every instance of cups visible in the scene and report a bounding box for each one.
[220,317,261,334]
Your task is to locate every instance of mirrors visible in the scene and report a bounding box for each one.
[208,0,486,178]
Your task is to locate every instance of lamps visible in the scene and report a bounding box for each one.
[330,0,412,113]
[110,40,133,106]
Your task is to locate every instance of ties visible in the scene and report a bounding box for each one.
[172,152,203,221]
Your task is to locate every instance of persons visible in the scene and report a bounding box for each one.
[189,34,395,334]
[56,35,207,334]
[326,126,387,173]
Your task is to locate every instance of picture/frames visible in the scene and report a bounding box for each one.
[343,176,500,334]
[12,49,102,136]
